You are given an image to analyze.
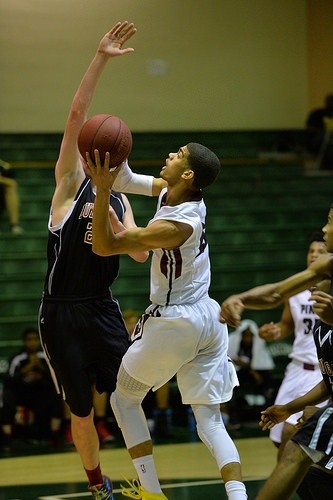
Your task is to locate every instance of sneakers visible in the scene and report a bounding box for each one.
[117,473,168,500]
[86,475,114,500]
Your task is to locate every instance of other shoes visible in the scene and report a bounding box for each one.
[66,425,76,445]
[93,422,114,443]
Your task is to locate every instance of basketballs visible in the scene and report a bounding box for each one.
[77,113,133,168]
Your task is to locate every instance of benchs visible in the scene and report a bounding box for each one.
[0,129,332,350]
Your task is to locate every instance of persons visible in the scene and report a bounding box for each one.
[35,16,152,500]
[0,308,280,441]
[218,198,333,500]
[75,141,253,500]
